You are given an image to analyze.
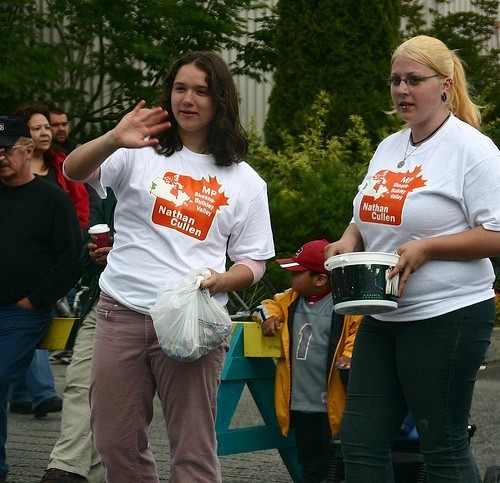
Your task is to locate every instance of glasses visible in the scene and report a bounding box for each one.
[386,75,438,85]
[5,142,30,153]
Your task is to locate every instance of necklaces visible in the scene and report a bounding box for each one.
[411,112,451,147]
[396,133,426,169]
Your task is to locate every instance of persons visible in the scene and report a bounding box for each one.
[47,183,104,364]
[323,34,500,482]
[60,50,275,483]
[0,104,89,420]
[249,240,370,483]
[38,184,117,483]
[33,108,103,226]
[0,116,84,483]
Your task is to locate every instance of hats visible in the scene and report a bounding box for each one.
[0,116,32,150]
[277,239,332,274]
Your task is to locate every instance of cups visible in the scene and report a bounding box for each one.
[88,223,110,251]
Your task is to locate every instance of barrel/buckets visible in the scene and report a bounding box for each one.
[324,252,400,314]
[36,318,81,351]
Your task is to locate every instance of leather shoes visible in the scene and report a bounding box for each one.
[35,396,65,417]
[9,402,35,415]
